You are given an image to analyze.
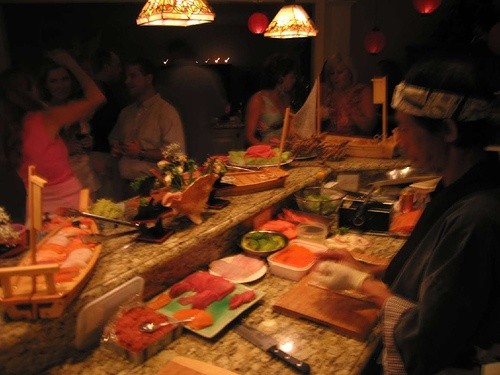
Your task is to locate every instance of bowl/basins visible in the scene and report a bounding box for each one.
[239,229,328,282]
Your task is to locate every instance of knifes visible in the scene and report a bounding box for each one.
[228,322,312,375]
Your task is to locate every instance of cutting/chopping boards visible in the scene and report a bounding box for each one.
[273,254,397,341]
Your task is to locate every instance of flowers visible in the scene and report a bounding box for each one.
[155,143,228,188]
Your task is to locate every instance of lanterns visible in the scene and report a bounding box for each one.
[364,27,387,53]
[412,0,441,14]
[248,11,270,34]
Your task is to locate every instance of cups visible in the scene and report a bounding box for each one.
[296,221,327,245]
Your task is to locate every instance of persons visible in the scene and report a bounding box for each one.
[321,54,500,375]
[0,40,299,219]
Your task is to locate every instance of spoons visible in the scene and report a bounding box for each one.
[141,318,193,332]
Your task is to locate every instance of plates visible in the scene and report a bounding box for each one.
[0,223,25,259]
[225,139,322,168]
[209,257,268,284]
[144,268,267,341]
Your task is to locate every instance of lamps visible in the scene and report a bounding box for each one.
[264,0,318,38]
[135,0,216,27]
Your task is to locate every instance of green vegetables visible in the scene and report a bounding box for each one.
[227,148,292,165]
[244,232,285,252]
[299,194,337,214]
